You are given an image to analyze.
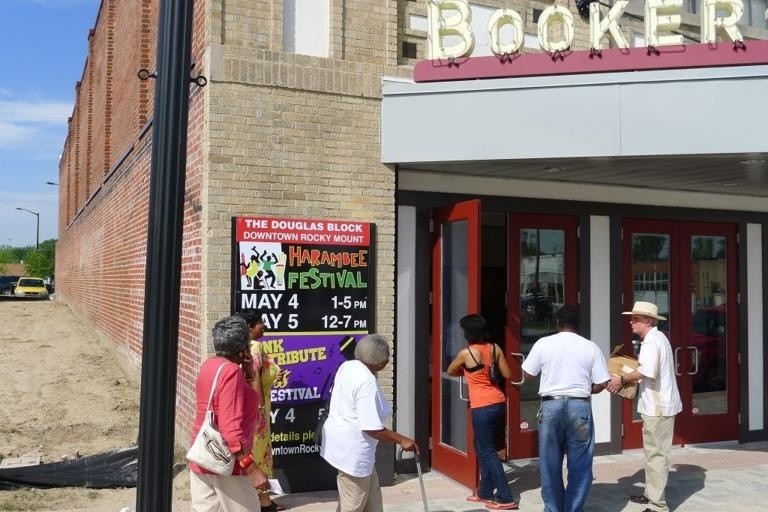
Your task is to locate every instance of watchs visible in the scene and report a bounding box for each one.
[620,375,627,386]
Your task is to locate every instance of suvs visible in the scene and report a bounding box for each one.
[0,276,55,295]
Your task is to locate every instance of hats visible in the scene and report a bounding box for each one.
[620,301,668,321]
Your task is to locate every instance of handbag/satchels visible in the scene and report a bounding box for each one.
[489,363,505,390]
[186,411,236,478]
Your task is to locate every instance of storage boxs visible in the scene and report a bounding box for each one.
[604,343,641,402]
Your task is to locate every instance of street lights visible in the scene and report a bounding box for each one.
[16,207,40,251]
[47,182,59,185]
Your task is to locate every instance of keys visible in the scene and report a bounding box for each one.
[535,407,543,424]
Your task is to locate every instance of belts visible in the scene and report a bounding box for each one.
[542,396,590,403]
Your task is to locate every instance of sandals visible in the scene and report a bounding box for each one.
[261,501,285,512]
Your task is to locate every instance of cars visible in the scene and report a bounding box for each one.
[13,276,49,298]
[662,303,726,390]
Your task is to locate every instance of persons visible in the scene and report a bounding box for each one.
[606,300,684,512]
[239,244,280,289]
[520,304,612,512]
[184,313,272,511]
[445,313,519,511]
[237,308,287,511]
[317,333,421,512]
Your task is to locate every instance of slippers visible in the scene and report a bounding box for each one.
[466,495,494,502]
[485,501,517,510]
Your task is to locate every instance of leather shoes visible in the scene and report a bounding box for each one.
[630,495,649,504]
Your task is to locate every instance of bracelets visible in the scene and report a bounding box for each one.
[237,454,255,469]
